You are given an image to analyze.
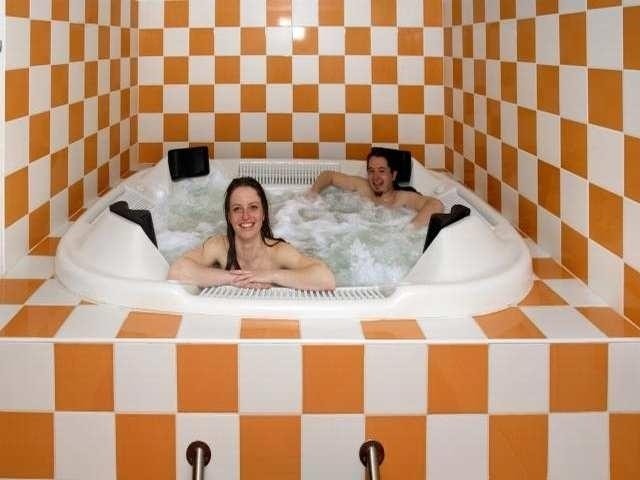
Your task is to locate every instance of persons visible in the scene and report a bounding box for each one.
[167,177,335,290]
[304,148,444,232]
